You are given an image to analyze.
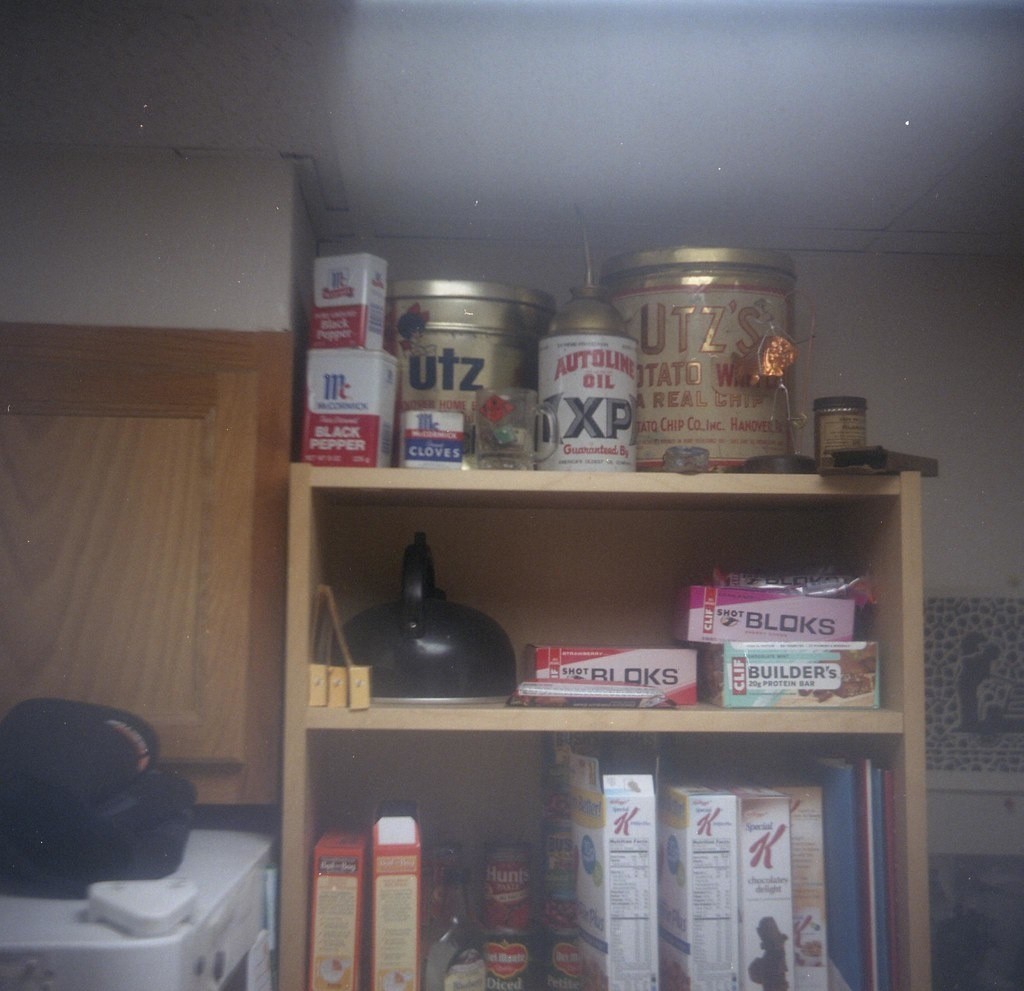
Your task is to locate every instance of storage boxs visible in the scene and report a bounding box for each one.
[674,585,855,643]
[308,800,421,991]
[701,643,879,710]
[572,753,830,990]
[524,642,697,707]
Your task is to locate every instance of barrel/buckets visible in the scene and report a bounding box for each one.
[383,275,557,470]
[597,245,798,469]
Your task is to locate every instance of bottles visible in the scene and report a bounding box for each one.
[811,394,870,472]
[537,286,639,474]
[417,813,582,991]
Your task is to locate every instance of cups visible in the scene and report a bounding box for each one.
[472,384,560,471]
[658,444,712,475]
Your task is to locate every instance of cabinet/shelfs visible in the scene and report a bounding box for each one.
[281,461,933,991]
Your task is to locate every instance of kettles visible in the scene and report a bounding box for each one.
[340,529,518,711]
